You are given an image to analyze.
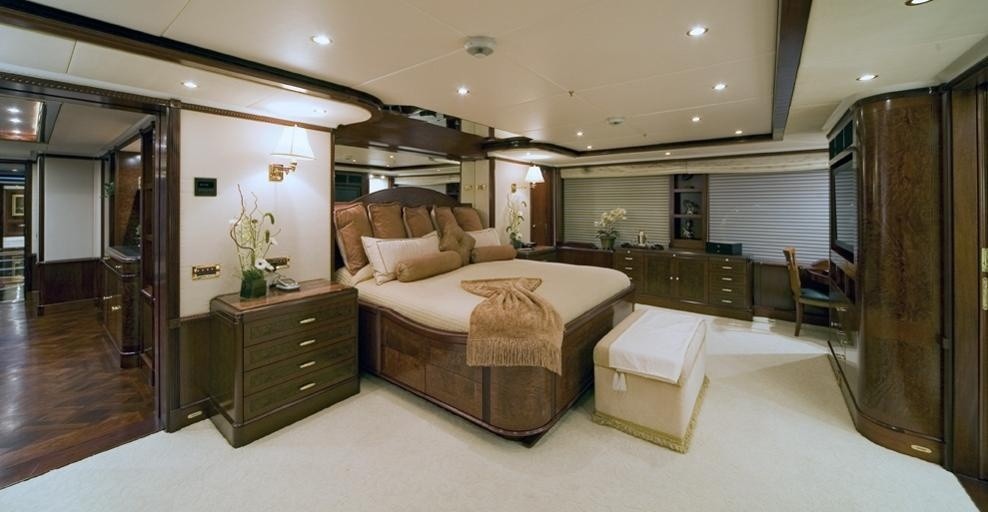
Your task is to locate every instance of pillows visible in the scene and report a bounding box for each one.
[333,200,517,284]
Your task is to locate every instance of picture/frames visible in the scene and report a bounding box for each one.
[11,194,24,216]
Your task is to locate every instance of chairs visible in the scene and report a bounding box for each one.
[782,247,829,336]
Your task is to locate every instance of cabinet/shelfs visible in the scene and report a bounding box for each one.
[708,254,754,323]
[645,251,707,314]
[668,173,707,253]
[103,258,139,369]
[754,258,799,320]
[136,121,157,384]
[208,279,360,447]
[612,246,644,304]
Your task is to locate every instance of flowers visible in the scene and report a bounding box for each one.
[504,193,527,238]
[226,183,282,279]
[592,207,628,238]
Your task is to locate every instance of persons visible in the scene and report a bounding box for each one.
[680,217,698,239]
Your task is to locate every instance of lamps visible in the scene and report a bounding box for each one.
[268,126,315,182]
[511,164,545,193]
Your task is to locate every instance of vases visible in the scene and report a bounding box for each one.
[240,279,267,299]
[599,236,616,250]
[511,238,521,248]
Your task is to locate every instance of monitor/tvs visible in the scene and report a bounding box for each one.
[831,159,854,263]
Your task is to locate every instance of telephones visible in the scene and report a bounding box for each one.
[275,277,300,292]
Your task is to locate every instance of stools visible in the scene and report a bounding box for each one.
[593,307,710,454]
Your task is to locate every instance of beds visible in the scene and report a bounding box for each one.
[335,185,635,447]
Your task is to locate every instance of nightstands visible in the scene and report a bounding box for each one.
[518,245,557,262]
